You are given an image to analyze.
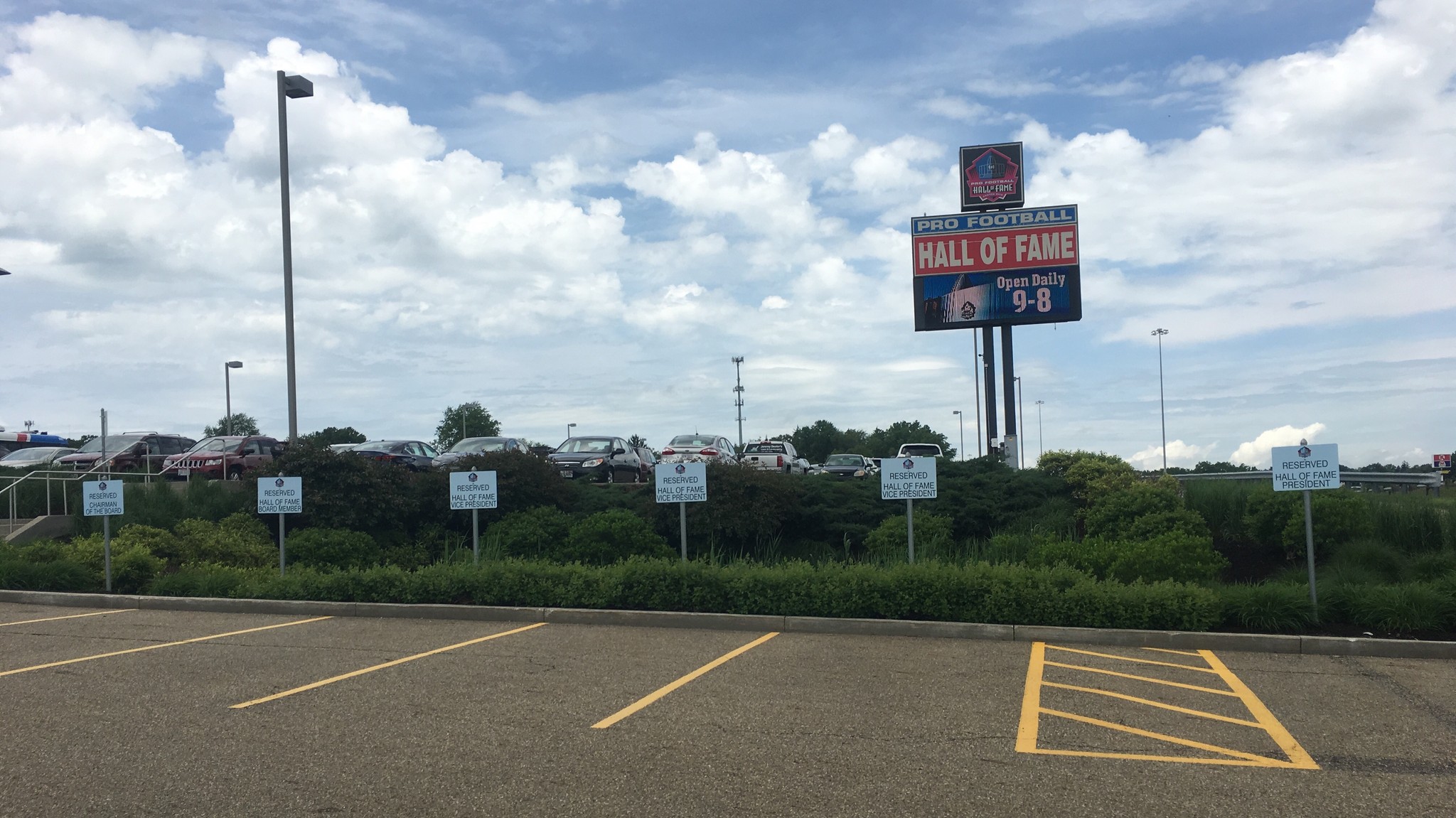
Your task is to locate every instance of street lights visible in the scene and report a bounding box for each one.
[568,423,577,439]
[1013,376,1024,470]
[953,411,963,461]
[462,404,475,439]
[1150,328,1169,474]
[1035,400,1045,461]
[225,361,243,436]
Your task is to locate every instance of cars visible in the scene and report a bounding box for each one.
[632,447,661,483]
[321,443,359,455]
[0,447,78,469]
[818,454,872,481]
[803,456,883,475]
[432,437,538,472]
[889,443,943,458]
[660,434,745,466]
[344,439,441,473]
[544,435,642,484]
[0,445,11,459]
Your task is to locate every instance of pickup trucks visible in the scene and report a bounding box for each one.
[740,441,808,475]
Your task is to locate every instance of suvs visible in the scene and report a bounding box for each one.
[162,434,282,481]
[51,432,198,479]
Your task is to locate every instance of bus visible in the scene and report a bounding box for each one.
[0,425,69,452]
